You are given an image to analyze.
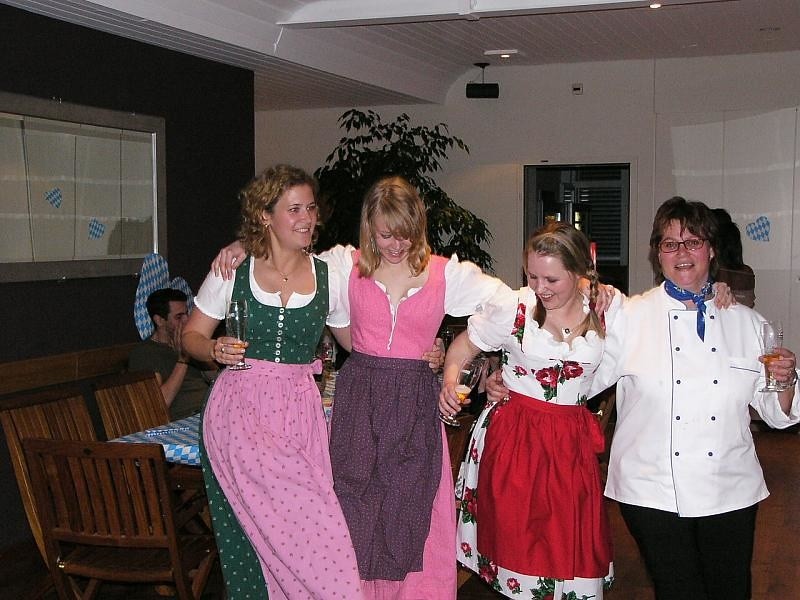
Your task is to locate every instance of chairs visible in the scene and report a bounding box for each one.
[446,412,475,487]
[0,370,218,600]
[597,389,639,556]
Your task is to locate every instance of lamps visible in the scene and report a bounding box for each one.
[466,63,499,99]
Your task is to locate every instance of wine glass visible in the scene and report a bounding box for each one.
[225,298,252,372]
[438,357,483,429]
[759,320,785,392]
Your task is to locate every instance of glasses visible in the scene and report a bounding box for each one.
[658,238,711,253]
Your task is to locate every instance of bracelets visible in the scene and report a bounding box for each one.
[176,360,189,365]
[774,370,798,388]
[213,340,216,359]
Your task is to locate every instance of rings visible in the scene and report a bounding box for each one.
[221,346,225,353]
[565,328,570,334]
[717,285,726,290]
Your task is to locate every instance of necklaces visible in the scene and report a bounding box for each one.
[280,271,288,289]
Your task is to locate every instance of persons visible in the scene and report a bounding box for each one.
[485,195,800,600]
[181,165,445,600]
[129,289,216,423]
[439,222,737,600]
[210,177,614,600]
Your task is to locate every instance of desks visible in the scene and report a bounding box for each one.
[103,411,206,491]
